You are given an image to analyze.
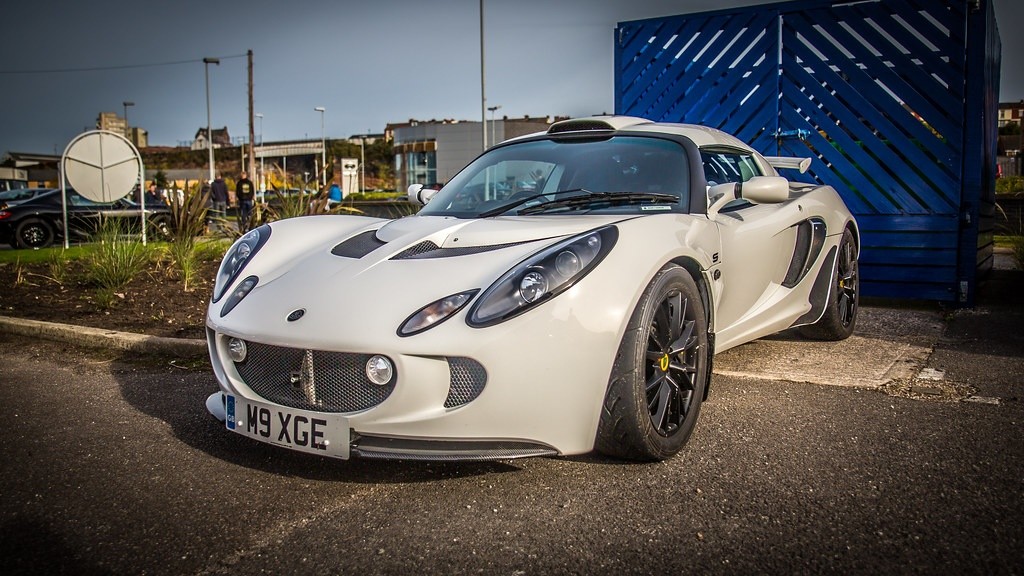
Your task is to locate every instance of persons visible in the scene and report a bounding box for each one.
[235,172,255,228]
[328,180,343,209]
[201,173,230,233]
[147,183,161,201]
[535,169,545,193]
[134,185,140,201]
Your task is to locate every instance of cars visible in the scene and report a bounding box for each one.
[0,188,173,250]
[461,181,536,199]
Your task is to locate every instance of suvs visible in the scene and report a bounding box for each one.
[256,188,312,197]
[159,190,185,208]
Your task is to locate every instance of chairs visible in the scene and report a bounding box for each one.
[660,152,688,197]
[564,160,628,197]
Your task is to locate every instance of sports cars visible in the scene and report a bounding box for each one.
[204,114,860,463]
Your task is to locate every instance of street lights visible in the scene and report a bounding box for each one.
[487,105,502,201]
[314,106,326,186]
[202,57,220,184]
[254,113,264,203]
[123,101,135,138]
